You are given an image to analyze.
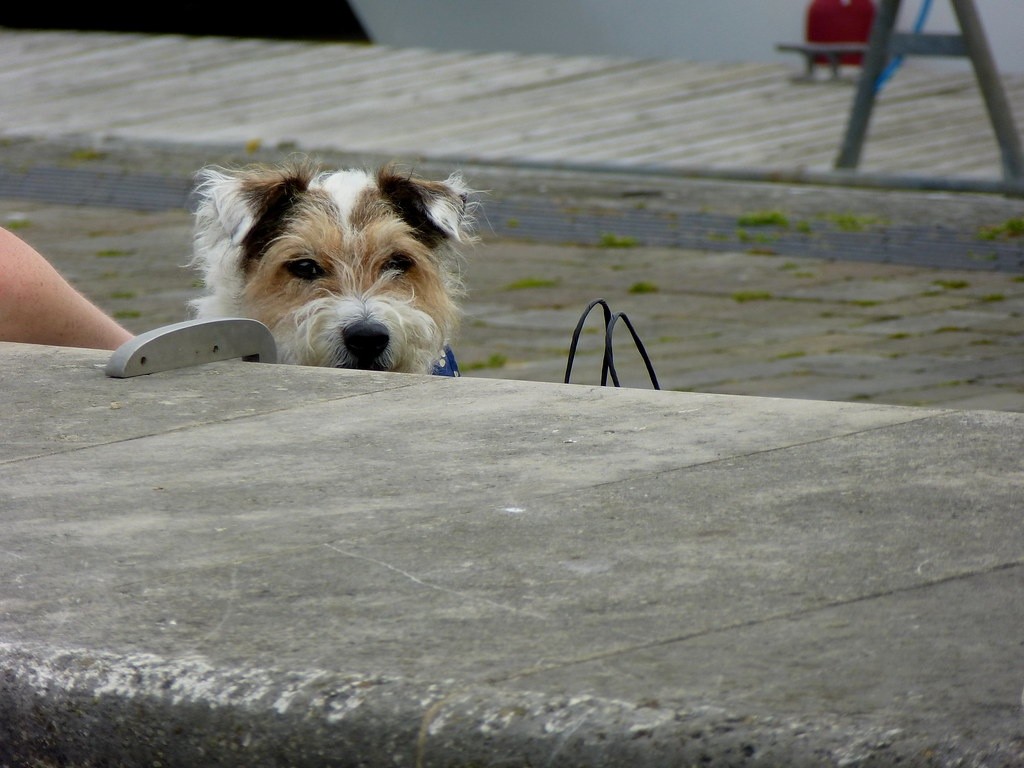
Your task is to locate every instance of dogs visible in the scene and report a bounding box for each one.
[178,153,498,377]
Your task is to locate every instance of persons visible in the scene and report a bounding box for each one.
[1,226,135,350]
